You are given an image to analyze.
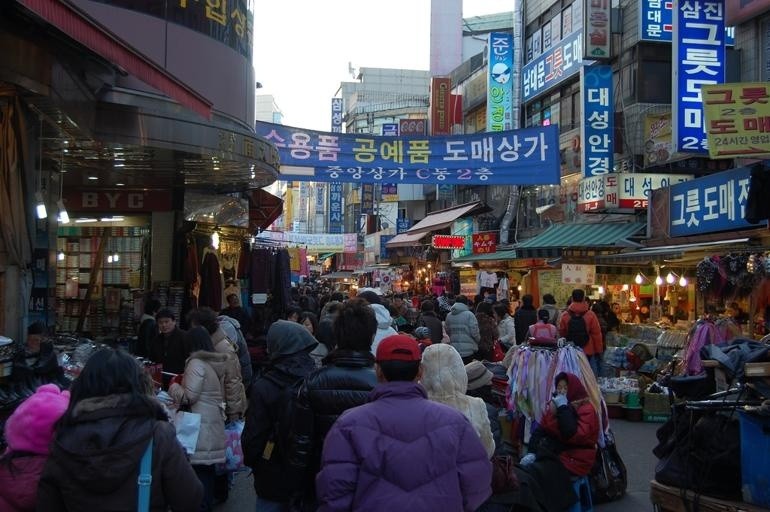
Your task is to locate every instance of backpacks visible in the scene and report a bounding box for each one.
[566,308,590,347]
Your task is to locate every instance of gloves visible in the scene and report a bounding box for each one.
[551,391,569,408]
[519,452,537,466]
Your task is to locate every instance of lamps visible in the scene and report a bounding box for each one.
[56,200,70,225]
[108,251,112,263]
[114,249,119,262]
[59,248,67,261]
[34,190,46,218]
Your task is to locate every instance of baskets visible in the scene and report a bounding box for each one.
[642,384,672,418]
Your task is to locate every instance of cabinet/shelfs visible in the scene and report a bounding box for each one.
[56,227,147,333]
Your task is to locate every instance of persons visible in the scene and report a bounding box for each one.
[638,305,652,324]
[726,303,751,324]
[239,270,626,512]
[2,294,261,512]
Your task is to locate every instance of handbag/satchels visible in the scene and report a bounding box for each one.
[213,420,252,476]
[490,338,505,363]
[168,391,202,456]
[491,454,519,494]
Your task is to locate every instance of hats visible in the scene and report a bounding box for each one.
[376,334,423,363]
[465,359,495,391]
[415,326,432,339]
[3,382,73,457]
